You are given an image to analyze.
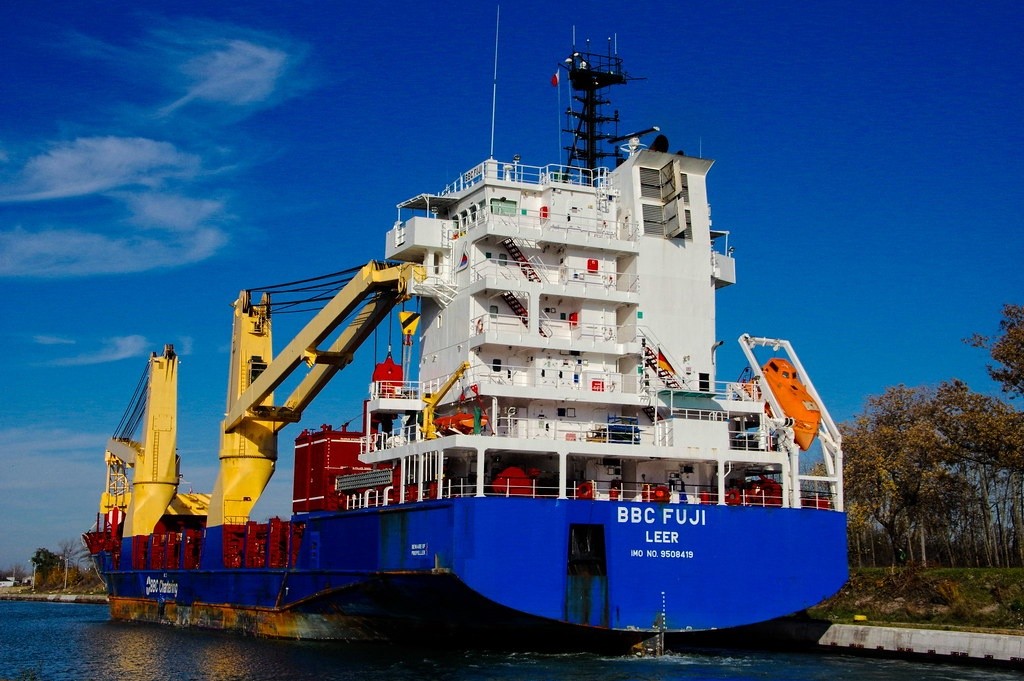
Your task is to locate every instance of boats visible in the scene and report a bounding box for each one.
[78,27,852,645]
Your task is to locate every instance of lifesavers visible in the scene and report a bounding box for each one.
[576,482,593,498]
[476,318,484,333]
[653,486,670,502]
[725,489,740,504]
